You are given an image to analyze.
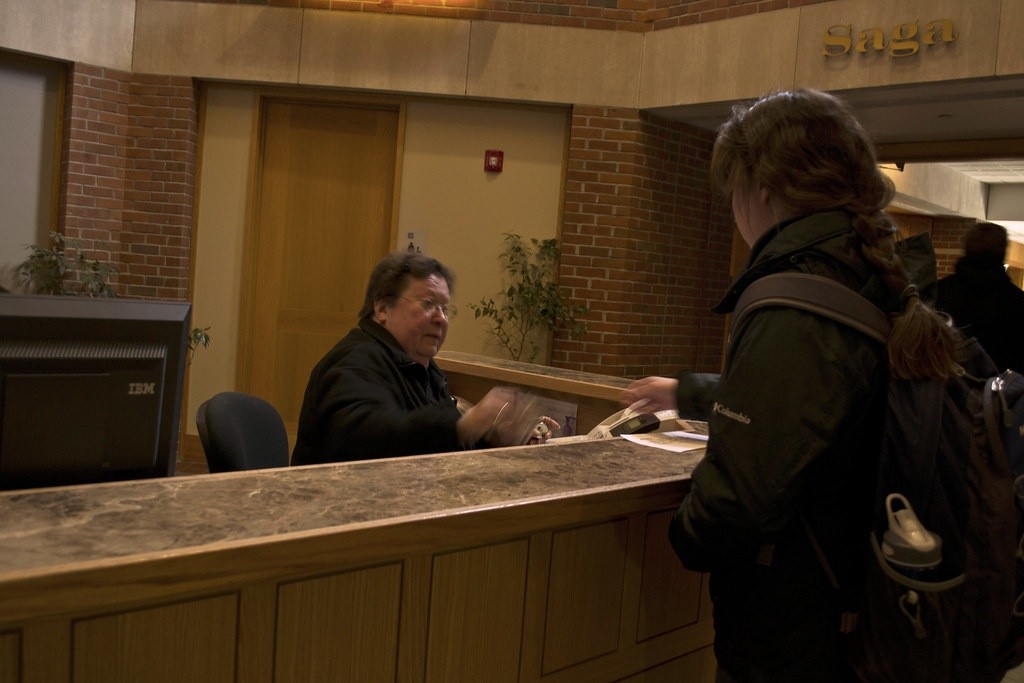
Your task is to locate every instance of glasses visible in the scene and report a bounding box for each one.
[394,291,457,320]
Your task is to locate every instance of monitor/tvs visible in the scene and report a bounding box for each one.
[0,291,194,498]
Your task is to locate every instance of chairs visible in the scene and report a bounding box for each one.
[196,392,289,475]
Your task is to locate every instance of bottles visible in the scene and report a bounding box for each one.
[880,493,944,582]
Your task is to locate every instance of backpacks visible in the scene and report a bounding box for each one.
[730,272,1024,683]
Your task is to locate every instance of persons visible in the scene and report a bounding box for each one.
[619,90,1024,683]
[290,252,561,466]
[919,222,1024,375]
